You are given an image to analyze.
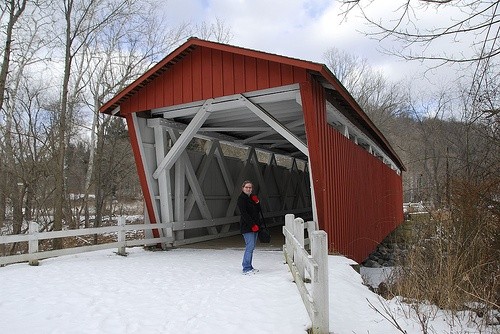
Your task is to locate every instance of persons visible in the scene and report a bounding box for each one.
[237,179,262,276]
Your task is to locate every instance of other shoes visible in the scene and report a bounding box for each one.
[242,268,259,276]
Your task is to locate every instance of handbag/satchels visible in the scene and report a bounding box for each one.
[259,226,271,243]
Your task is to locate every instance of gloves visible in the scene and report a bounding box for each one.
[252,225,259,232]
[252,195,259,204]
